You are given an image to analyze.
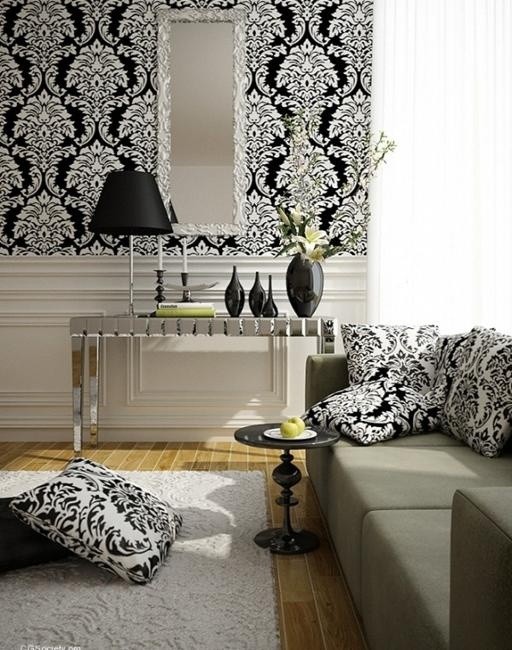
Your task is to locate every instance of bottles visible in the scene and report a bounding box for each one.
[225,265,279,318]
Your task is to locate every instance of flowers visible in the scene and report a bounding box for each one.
[218,370,219,372]
[273,205,332,263]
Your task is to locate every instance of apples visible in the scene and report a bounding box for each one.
[280,416,305,438]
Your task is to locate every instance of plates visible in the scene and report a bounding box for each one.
[263,428,319,441]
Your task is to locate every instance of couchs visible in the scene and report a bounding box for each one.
[307,354,512,649]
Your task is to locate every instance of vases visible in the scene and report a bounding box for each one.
[261,273,278,317]
[225,265,245,317]
[283,254,324,317]
[248,270,267,318]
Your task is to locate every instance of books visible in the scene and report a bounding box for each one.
[155,299,218,317]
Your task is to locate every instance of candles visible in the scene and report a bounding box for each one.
[182,240,190,273]
[157,236,163,270]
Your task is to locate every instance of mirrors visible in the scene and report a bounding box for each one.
[156,9,246,236]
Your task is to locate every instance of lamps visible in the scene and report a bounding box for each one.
[88,172,173,317]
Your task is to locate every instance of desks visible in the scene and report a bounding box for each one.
[234,424,341,555]
[70,317,338,453]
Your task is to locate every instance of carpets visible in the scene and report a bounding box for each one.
[0,470,280,650]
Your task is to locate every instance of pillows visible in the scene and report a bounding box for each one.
[10,457,183,585]
[301,379,446,444]
[340,324,439,395]
[438,325,512,458]
[423,327,496,405]
[0,498,68,571]
